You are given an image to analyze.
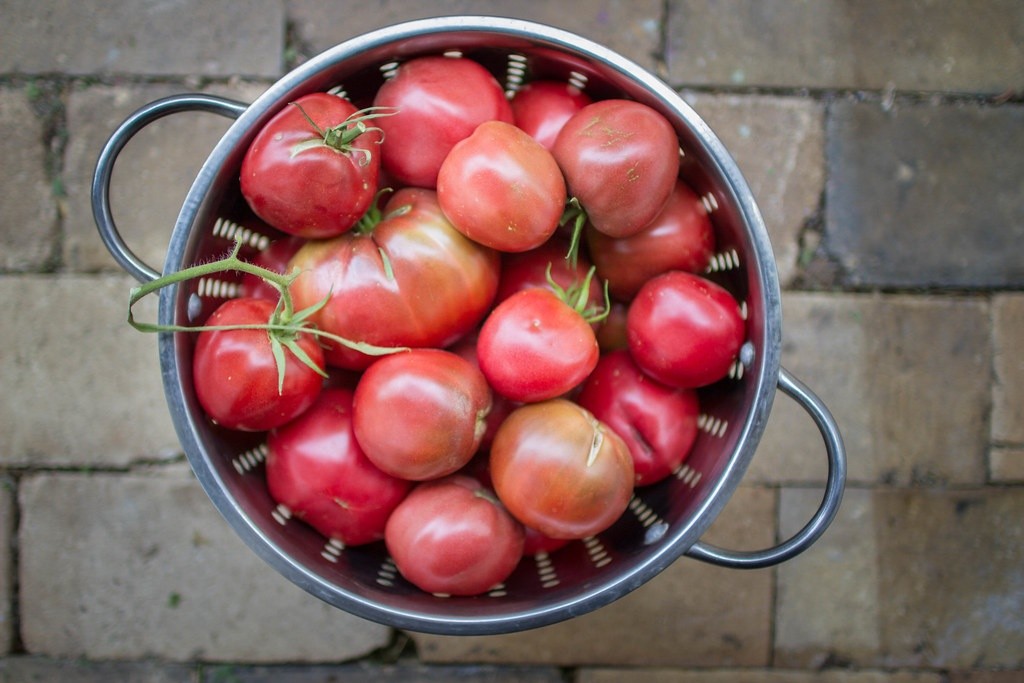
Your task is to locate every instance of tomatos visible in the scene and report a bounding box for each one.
[193,57,743,593]
